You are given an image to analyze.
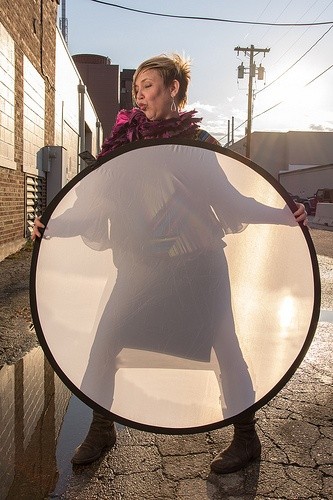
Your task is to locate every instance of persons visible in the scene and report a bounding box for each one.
[31,54,314,475]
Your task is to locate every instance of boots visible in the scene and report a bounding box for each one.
[71,408,117,464]
[209,418,263,473]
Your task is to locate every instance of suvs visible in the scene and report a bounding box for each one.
[303,188,333,215]
